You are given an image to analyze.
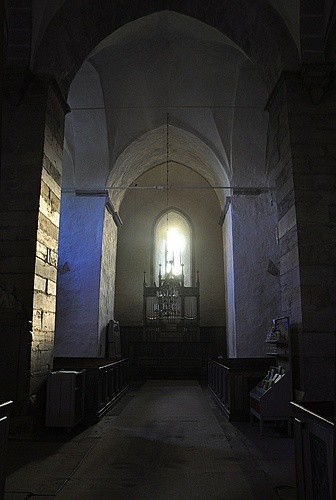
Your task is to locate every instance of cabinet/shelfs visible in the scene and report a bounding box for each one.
[249,317,294,437]
